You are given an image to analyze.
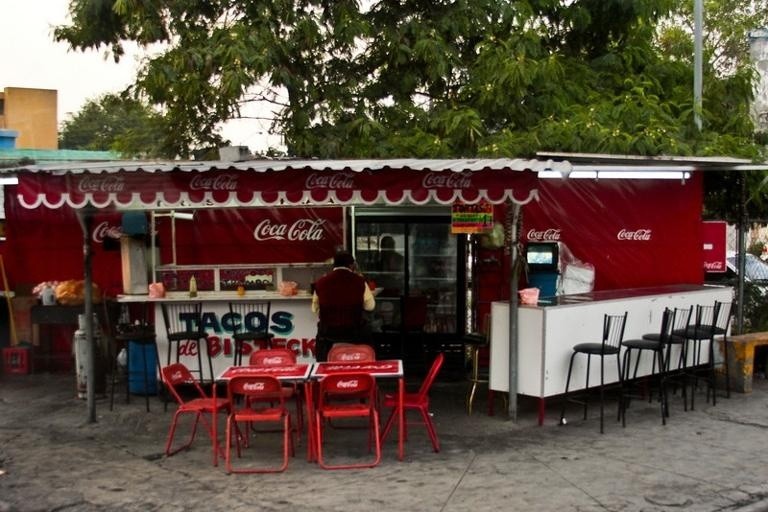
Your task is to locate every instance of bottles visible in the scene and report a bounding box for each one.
[190,274,197,297]
[42,285,57,305]
[411,226,457,333]
[309,274,315,294]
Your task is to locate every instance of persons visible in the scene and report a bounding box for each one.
[311,251,376,404]
[378,235,404,271]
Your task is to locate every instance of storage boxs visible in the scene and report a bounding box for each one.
[0,346,29,374]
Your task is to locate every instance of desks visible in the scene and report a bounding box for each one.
[486,282,739,426]
[30,304,99,376]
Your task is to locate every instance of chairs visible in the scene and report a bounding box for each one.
[243,348,304,450]
[315,372,382,470]
[325,342,379,446]
[658,300,719,412]
[225,375,295,477]
[379,352,445,456]
[161,300,213,412]
[161,363,246,467]
[673,297,736,406]
[104,298,169,412]
[627,305,693,417]
[228,301,273,404]
[559,310,627,435]
[617,308,671,425]
[462,313,508,416]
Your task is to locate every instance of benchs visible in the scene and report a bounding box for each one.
[714,332,768,394]
